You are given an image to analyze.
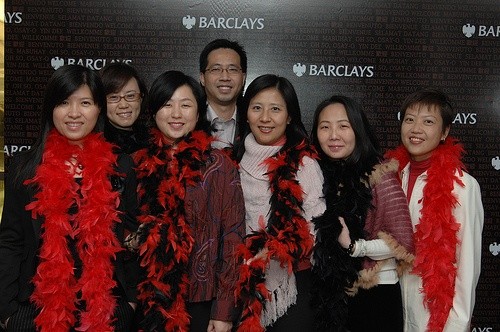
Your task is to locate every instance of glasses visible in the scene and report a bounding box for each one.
[205,66,243,74]
[106,93,143,103]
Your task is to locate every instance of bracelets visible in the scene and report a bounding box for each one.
[347,240,355,254]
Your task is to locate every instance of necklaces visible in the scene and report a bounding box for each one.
[130,128,216,332]
[23,127,125,332]
[312,152,375,304]
[226,134,317,332]
[384,137,468,332]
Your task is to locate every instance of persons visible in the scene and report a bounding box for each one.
[226,74,327,332]
[197,38,247,149]
[0,65,137,332]
[309,94,415,332]
[94,61,148,149]
[125,71,245,332]
[379,86,500,332]
